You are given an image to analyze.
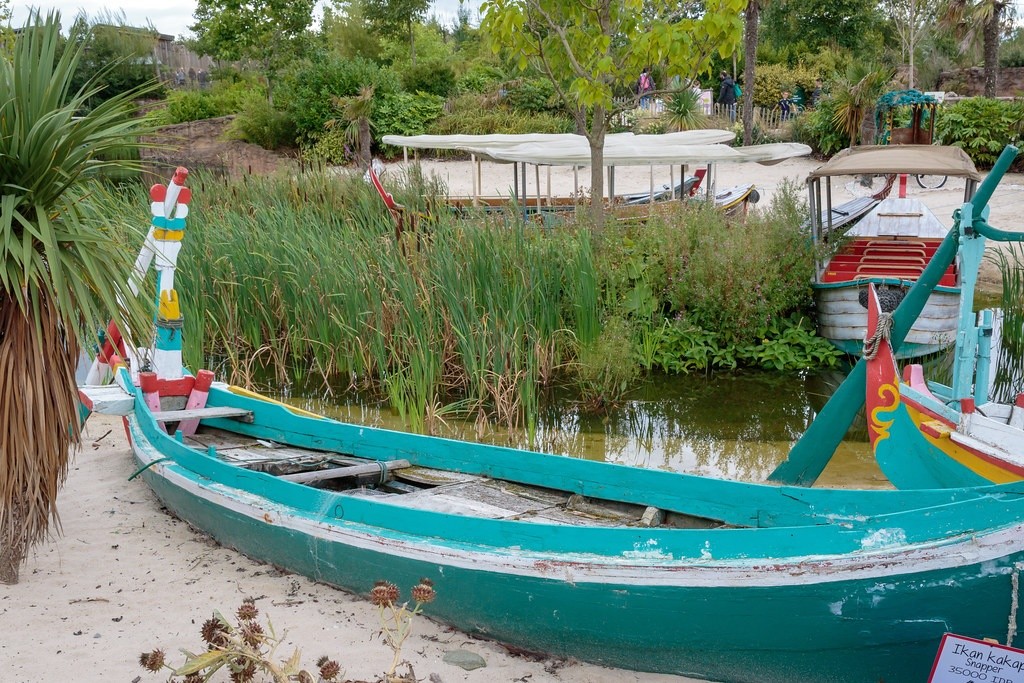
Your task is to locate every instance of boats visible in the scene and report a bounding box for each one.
[804,144,981,360]
[68,141,1024,683]
[379,127,813,225]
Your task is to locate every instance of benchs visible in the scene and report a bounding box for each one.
[151,406,251,422]
[360,480,677,531]
[170,431,335,467]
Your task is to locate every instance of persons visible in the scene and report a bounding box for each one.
[772,92,793,125]
[715,70,743,120]
[639,68,656,111]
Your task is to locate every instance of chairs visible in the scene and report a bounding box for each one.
[825,238,955,287]
